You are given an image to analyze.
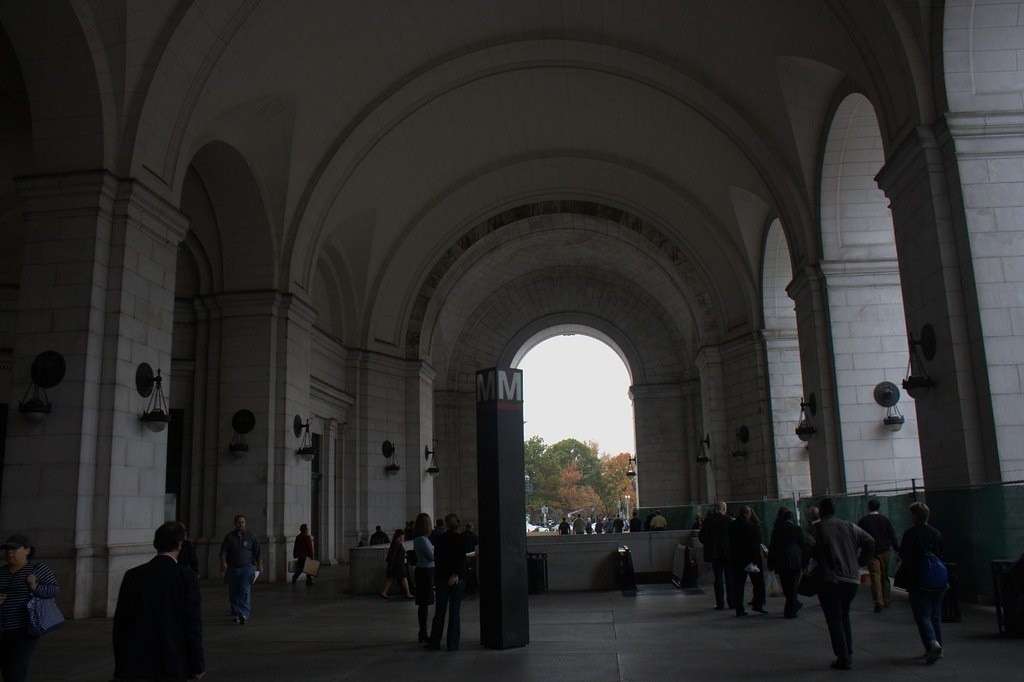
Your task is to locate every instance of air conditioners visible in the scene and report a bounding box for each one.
[382,440,401,476]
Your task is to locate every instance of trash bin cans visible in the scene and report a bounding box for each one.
[526,551,549,594]
[989,559,1024,635]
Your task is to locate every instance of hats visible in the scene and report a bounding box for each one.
[695,513,704,520]
[0,534,31,549]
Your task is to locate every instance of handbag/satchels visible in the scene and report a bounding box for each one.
[406,550,417,566]
[893,523,950,593]
[764,555,784,598]
[798,565,832,598]
[24,561,64,635]
[302,556,320,576]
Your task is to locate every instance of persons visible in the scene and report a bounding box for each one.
[367,519,480,598]
[431,513,469,651]
[805,496,877,671]
[107,522,208,682]
[0,523,63,682]
[549,508,669,535]
[287,523,319,585]
[895,500,951,664]
[406,510,436,641]
[222,514,263,626]
[858,499,897,612]
[698,499,816,620]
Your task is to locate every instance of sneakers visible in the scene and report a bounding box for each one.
[923,638,944,665]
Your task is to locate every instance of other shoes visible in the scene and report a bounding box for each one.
[232,614,247,625]
[747,599,766,605]
[831,660,852,670]
[875,604,882,613]
[752,605,768,613]
[424,644,440,650]
[713,605,724,610]
[736,611,747,617]
[404,594,414,599]
[305,579,313,584]
[380,594,391,600]
[292,575,296,585]
[448,647,458,651]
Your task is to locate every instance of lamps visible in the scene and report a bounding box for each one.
[135,361,171,433]
[228,409,256,459]
[626,454,636,479]
[294,414,317,462]
[425,444,439,477]
[900,324,939,400]
[697,432,710,467]
[17,350,67,427]
[874,380,905,432]
[732,425,749,463]
[796,391,817,442]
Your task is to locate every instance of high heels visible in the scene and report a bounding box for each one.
[418,627,429,642]
